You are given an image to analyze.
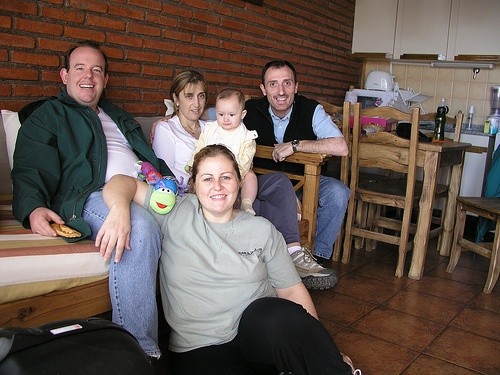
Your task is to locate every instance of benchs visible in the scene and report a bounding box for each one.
[1,108,325,333]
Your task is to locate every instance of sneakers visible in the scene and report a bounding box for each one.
[288,247,332,277]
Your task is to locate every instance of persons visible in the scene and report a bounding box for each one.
[152,71,337,290]
[13,43,177,358]
[150,61,352,266]
[187,89,258,215]
[95,145,361,375]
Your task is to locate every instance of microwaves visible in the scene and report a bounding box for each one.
[351,86,411,113]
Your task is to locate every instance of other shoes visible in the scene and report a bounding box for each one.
[300,270,338,290]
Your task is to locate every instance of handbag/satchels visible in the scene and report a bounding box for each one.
[0,318,161,375]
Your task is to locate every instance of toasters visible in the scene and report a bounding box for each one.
[365,70,395,90]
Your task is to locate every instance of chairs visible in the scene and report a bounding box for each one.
[315,101,500,294]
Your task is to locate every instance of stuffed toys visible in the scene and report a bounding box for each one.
[138,161,178,214]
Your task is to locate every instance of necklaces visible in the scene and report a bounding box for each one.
[181,117,198,135]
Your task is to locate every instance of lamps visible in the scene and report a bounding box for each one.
[431,62,496,68]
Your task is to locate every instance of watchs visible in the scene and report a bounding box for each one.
[291,140,300,153]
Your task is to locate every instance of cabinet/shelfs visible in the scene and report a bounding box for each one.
[351,0,398,59]
[446,0,500,62]
[395,0,451,63]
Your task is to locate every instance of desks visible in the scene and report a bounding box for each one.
[336,134,471,283]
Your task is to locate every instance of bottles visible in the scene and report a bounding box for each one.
[432,97,449,143]
[484,121,490,133]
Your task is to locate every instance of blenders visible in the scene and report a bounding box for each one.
[485,85,500,135]
